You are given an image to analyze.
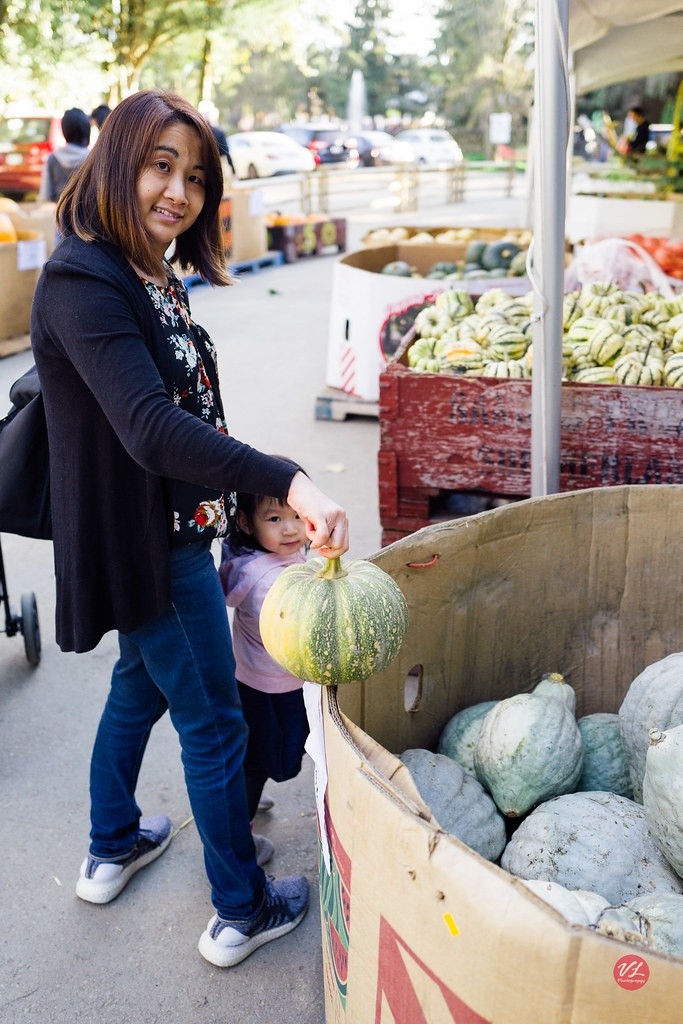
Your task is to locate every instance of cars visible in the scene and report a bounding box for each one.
[354,130,404,168]
[225,130,320,180]
[393,128,466,167]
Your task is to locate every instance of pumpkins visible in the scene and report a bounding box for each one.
[259,548,408,684]
[0,197,34,244]
[391,646,683,965]
[407,289,683,390]
[367,226,683,282]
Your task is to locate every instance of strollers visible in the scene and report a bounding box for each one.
[0,530,43,666]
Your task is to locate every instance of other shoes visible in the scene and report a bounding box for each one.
[258,792,275,812]
[252,833,275,866]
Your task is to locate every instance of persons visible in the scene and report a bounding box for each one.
[617,104,652,163]
[89,105,112,151]
[31,86,353,969]
[213,452,312,863]
[37,107,91,203]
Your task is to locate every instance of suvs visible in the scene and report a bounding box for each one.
[274,120,360,171]
[0,113,65,204]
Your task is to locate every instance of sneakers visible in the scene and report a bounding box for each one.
[76,815,172,904]
[199,873,310,967]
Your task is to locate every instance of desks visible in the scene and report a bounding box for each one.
[268,218,347,263]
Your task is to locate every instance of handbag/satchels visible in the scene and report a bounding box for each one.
[1,365,53,540]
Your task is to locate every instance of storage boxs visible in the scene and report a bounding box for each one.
[178,187,265,279]
[328,241,537,408]
[376,293,683,550]
[0,228,47,341]
[359,225,585,271]
[313,475,683,1024]
[14,216,56,254]
